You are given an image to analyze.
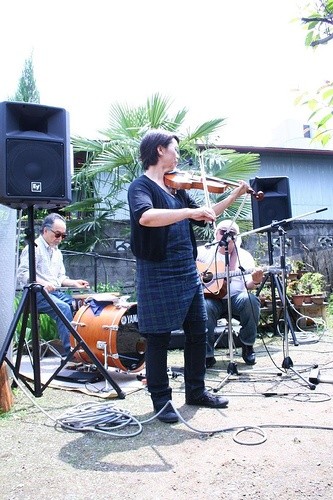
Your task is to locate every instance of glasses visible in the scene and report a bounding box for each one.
[50,230,67,240]
[220,230,236,236]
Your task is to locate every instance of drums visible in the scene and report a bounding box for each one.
[70,302,146,373]
[72,292,120,318]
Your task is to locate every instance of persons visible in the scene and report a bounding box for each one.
[128,131,248,422]
[197,220,263,367]
[18,213,90,357]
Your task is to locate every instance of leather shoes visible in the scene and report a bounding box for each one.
[206,358,216,368]
[242,344,256,365]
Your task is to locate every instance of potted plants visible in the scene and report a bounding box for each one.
[259,260,325,308]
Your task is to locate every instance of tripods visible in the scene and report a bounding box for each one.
[206,207,328,392]
[0,207,126,400]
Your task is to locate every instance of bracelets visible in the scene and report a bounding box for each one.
[254,283,260,285]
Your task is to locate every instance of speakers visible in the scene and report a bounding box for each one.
[250,177,292,233]
[0,101,71,210]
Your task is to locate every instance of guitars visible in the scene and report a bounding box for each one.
[195,260,292,299]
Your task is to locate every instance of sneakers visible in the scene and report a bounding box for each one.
[153,401,178,423]
[186,390,229,407]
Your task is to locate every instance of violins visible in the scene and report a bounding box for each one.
[164,167,264,200]
